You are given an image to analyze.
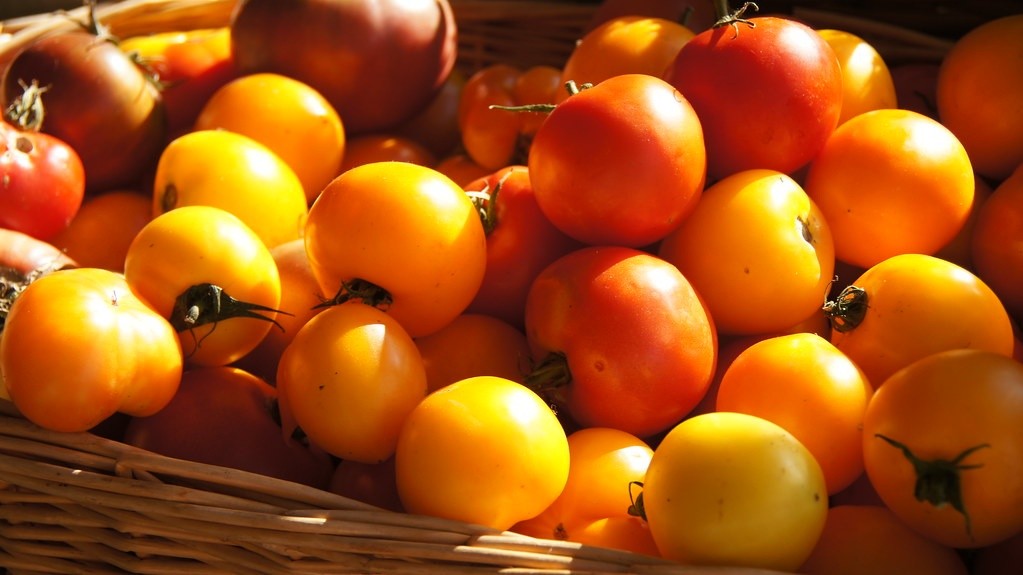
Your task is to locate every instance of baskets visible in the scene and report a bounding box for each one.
[0,0,959,575]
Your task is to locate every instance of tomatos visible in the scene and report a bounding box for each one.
[0,1,1023,573]
